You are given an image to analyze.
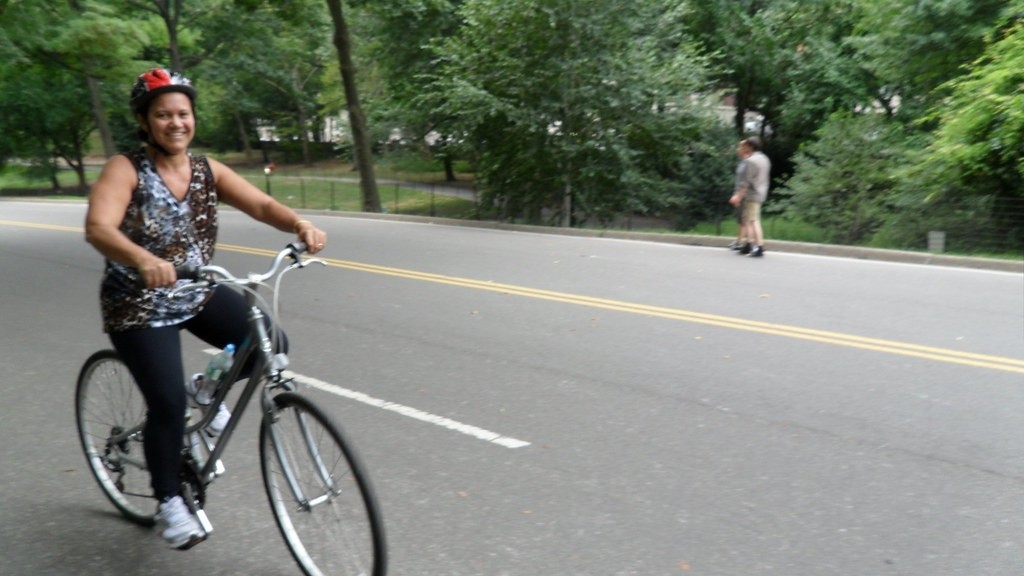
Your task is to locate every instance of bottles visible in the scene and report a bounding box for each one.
[196,344,238,405]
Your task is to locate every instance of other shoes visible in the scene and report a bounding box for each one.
[727,241,745,249]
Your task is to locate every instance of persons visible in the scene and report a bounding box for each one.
[86,66,327,552]
[727,138,774,258]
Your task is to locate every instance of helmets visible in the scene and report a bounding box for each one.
[129,67,198,114]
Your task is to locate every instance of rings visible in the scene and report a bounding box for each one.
[315,242,325,247]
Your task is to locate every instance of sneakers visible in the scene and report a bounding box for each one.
[751,246,764,257]
[741,244,752,253]
[184,372,232,438]
[153,494,208,551]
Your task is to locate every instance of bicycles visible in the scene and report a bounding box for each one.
[74,243,389,576]
